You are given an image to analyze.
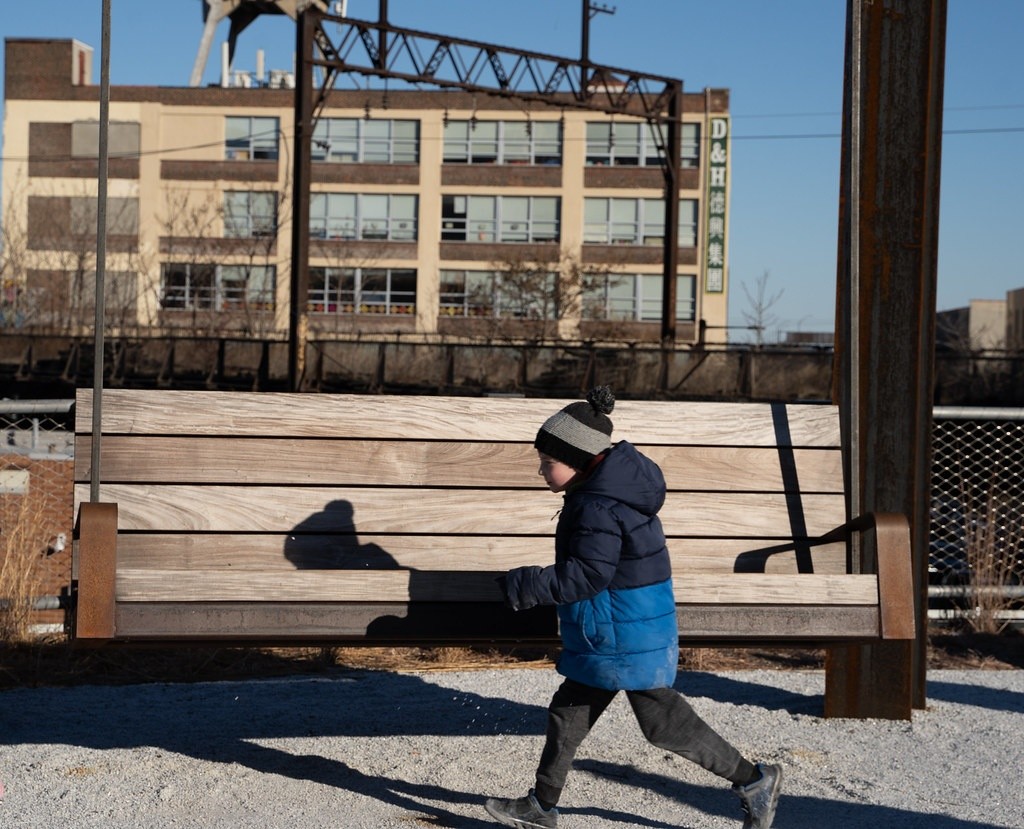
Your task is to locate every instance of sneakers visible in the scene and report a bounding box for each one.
[485,788,558,829]
[731,762,784,829]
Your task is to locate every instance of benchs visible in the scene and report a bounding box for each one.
[68,382,910,720]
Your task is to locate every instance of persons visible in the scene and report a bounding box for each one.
[483,382,784,828]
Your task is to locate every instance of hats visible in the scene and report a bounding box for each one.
[534,384,614,471]
